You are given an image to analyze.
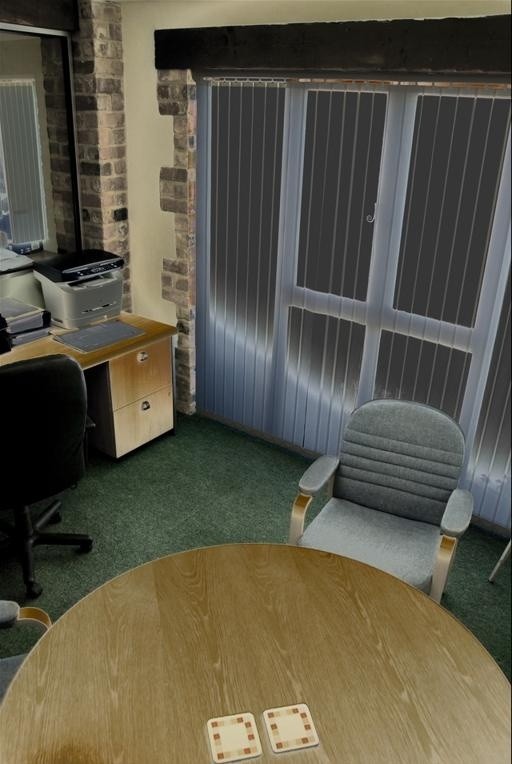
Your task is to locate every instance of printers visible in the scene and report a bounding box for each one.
[30,247,125,329]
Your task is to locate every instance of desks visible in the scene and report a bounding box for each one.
[0,312,178,461]
[1,543,512,763]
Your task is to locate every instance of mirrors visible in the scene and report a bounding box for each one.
[1,23,82,276]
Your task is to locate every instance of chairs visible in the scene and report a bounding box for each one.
[289,399,475,603]
[0,599,52,704]
[1,354,96,599]
